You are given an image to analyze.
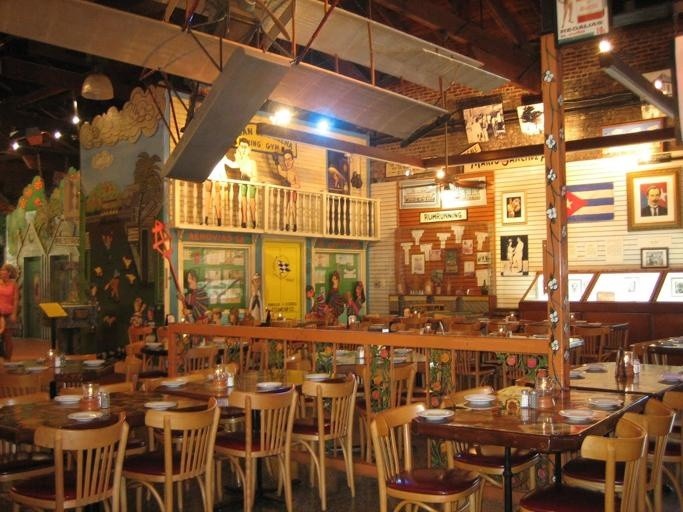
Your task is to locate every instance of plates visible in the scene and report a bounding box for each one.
[463,394,496,405]
[588,394,624,411]
[54,395,82,404]
[256,382,282,390]
[66,412,102,423]
[335,349,348,356]
[418,409,454,422]
[557,408,594,424]
[660,372,682,380]
[394,348,414,354]
[26,366,48,373]
[306,373,330,382]
[84,358,106,366]
[144,402,177,411]
[584,362,607,371]
[568,369,583,378]
[161,378,188,388]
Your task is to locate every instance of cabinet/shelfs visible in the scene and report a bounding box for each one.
[579,266,662,350]
[649,267,682,344]
[515,268,599,344]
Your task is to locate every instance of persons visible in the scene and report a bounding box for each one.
[92,270,366,354]
[641,186,667,217]
[506,239,515,272]
[273,149,297,232]
[507,198,515,217]
[0,264,20,360]
[513,237,524,272]
[205,154,240,226]
[562,0,574,28]
[221,138,257,229]
[466,110,506,143]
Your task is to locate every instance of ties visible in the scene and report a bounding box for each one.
[652,206,658,215]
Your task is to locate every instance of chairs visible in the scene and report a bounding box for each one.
[560,397,675,511]
[269,371,362,511]
[195,382,299,511]
[2,408,131,512]
[365,401,486,511]
[429,382,543,508]
[514,416,650,512]
[0,302,682,468]
[105,395,221,512]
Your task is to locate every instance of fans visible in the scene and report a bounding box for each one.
[399,75,492,192]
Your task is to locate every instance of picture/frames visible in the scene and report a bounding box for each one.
[625,165,682,232]
[498,188,527,225]
[408,237,491,280]
[639,247,668,268]
[396,180,439,210]
[596,115,665,157]
[440,175,488,209]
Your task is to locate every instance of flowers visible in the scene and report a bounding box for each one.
[540,62,569,369]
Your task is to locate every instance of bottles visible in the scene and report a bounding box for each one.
[44,346,67,367]
[206,364,234,390]
[356,344,390,361]
[615,345,640,379]
[79,381,111,411]
[519,369,554,409]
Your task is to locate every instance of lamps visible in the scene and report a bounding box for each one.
[78,53,115,101]
